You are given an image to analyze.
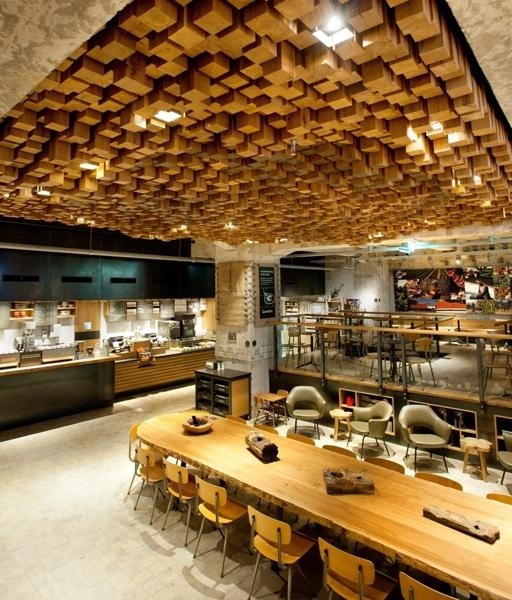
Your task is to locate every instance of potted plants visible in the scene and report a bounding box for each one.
[330,283,344,301]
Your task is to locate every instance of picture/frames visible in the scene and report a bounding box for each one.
[252,262,280,328]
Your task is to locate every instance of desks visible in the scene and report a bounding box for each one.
[456,313,512,335]
[139,415,511,600]
[329,312,454,352]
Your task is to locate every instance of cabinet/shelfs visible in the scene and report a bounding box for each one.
[49,253,102,303]
[100,257,145,299]
[185,262,214,298]
[326,296,361,347]
[146,259,184,298]
[0,248,50,301]
[281,296,324,369]
[195,368,251,419]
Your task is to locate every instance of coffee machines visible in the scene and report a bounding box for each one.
[175,311,195,338]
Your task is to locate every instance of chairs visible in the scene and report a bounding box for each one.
[267,432,315,507]
[430,431,436,432]
[392,332,419,381]
[487,492,512,506]
[247,505,319,600]
[313,329,342,371]
[193,475,254,579]
[496,429,512,486]
[407,470,463,572]
[397,572,458,600]
[234,424,278,495]
[205,414,246,480]
[362,330,389,379]
[315,537,398,600]
[354,455,404,553]
[286,326,305,369]
[344,330,361,358]
[483,366,512,393]
[398,404,451,473]
[287,386,327,441]
[127,422,143,495]
[405,339,437,386]
[306,445,356,527]
[161,457,199,547]
[487,330,512,376]
[349,401,393,460]
[265,390,289,421]
[135,447,169,526]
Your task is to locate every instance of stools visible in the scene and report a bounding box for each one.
[254,392,287,427]
[329,408,353,442]
[462,436,493,482]
